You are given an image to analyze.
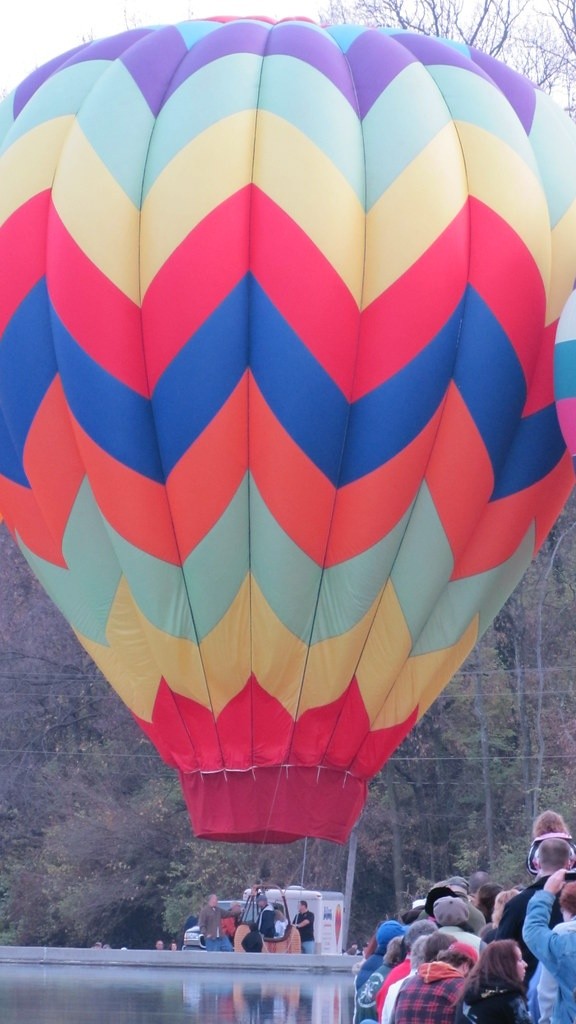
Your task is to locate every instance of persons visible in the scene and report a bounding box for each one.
[90,879,317,956]
[342,809,576,1024]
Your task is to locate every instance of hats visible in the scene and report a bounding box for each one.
[405,919,438,951]
[445,876,469,890]
[448,943,478,963]
[96,942,101,945]
[257,895,266,901]
[425,887,457,919]
[376,920,410,948]
[433,896,469,928]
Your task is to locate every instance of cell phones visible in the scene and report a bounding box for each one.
[564,872,576,881]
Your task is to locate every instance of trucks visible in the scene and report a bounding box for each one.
[183,883,344,956]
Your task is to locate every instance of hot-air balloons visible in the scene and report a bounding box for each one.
[0,15,576,845]
